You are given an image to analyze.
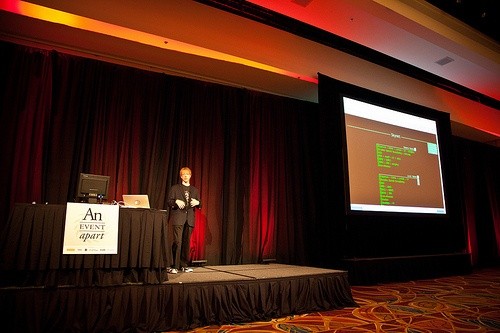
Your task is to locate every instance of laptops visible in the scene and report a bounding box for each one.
[122,195,150,208]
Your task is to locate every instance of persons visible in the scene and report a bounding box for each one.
[168,167,202,273]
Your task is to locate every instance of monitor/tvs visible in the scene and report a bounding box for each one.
[77,173,110,204]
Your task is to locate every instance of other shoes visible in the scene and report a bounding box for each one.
[181,267,194,273]
[170,268,181,274]
